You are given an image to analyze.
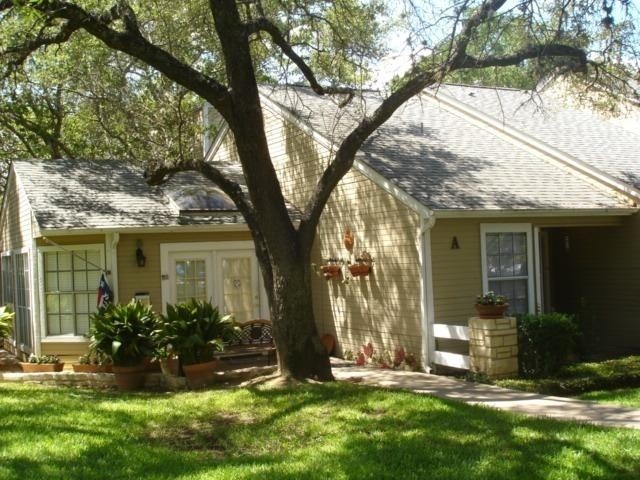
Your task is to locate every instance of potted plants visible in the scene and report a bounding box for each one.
[322,252,372,278]
[72,295,234,390]
[18,353,65,373]
[475,291,509,319]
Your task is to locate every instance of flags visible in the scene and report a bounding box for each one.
[97,272,113,313]
